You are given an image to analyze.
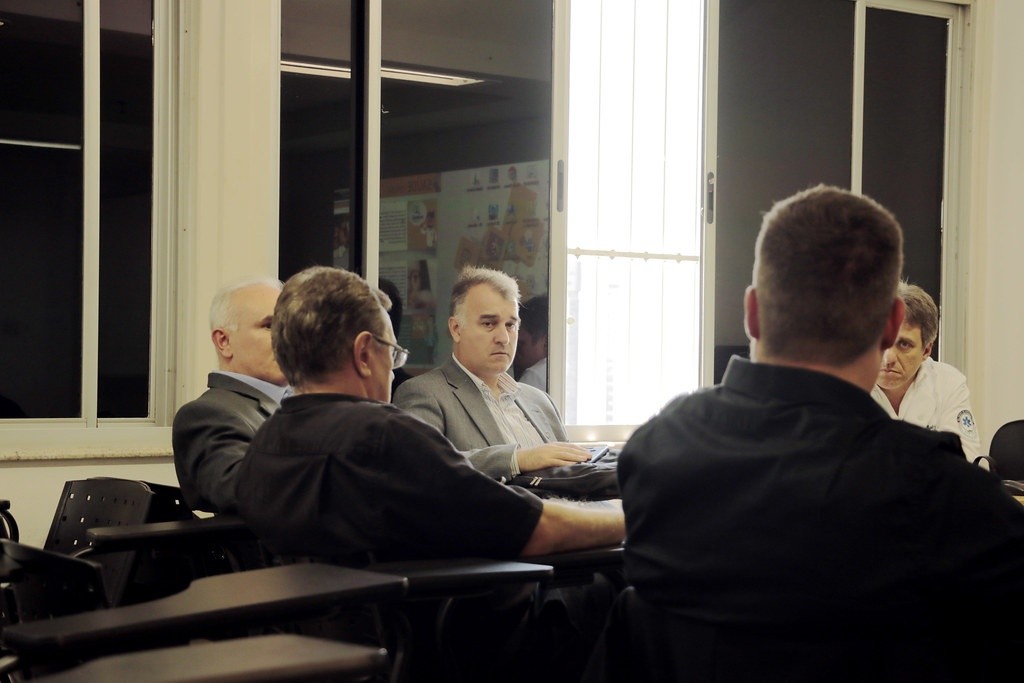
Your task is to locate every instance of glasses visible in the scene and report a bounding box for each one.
[373,335,410,369]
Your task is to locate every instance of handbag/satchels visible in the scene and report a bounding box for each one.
[504,446,622,500]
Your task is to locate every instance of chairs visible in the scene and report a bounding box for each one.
[0,420,1024,683]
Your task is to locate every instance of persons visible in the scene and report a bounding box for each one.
[392,266,593,477]
[171,276,296,508]
[505,298,548,392]
[871,282,981,462]
[242,266,631,683]
[616,187,1024,683]
